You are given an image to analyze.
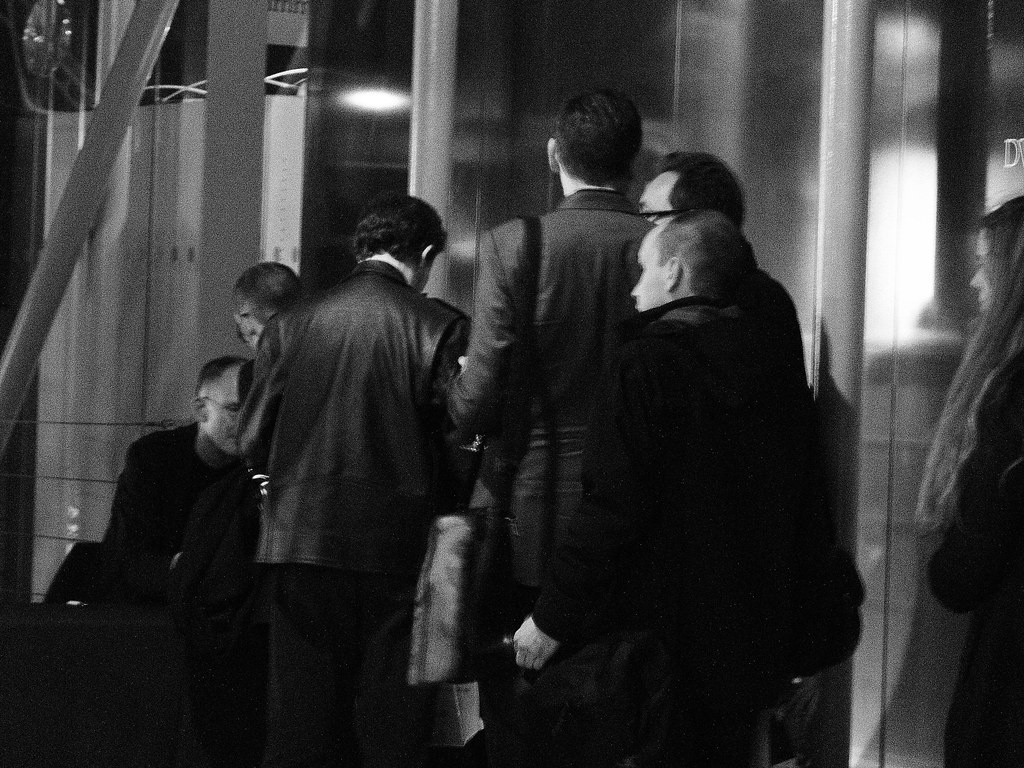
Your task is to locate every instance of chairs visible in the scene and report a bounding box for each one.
[0,602,212,768]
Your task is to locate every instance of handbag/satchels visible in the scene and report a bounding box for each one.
[408,513,514,683]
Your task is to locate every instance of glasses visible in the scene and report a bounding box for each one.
[201,396,240,420]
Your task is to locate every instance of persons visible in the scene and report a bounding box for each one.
[912,195,1024,768]
[97,89,867,768]
